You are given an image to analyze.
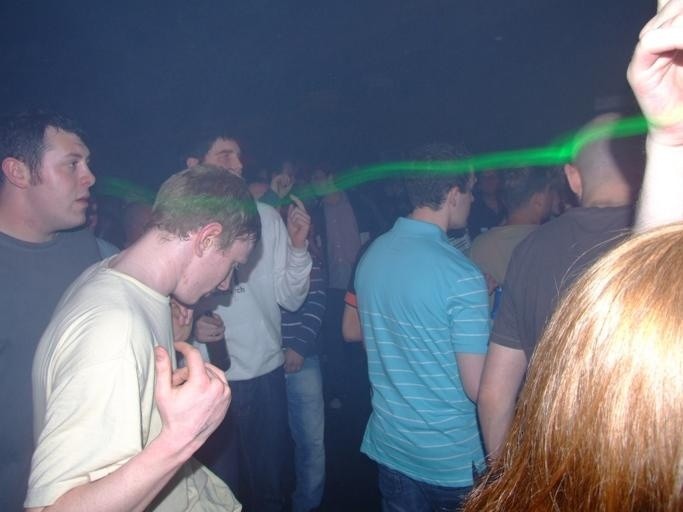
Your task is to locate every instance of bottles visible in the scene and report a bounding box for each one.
[204,311,231,372]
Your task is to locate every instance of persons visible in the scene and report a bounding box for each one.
[2,105,362,512]
[342,1,683,512]
